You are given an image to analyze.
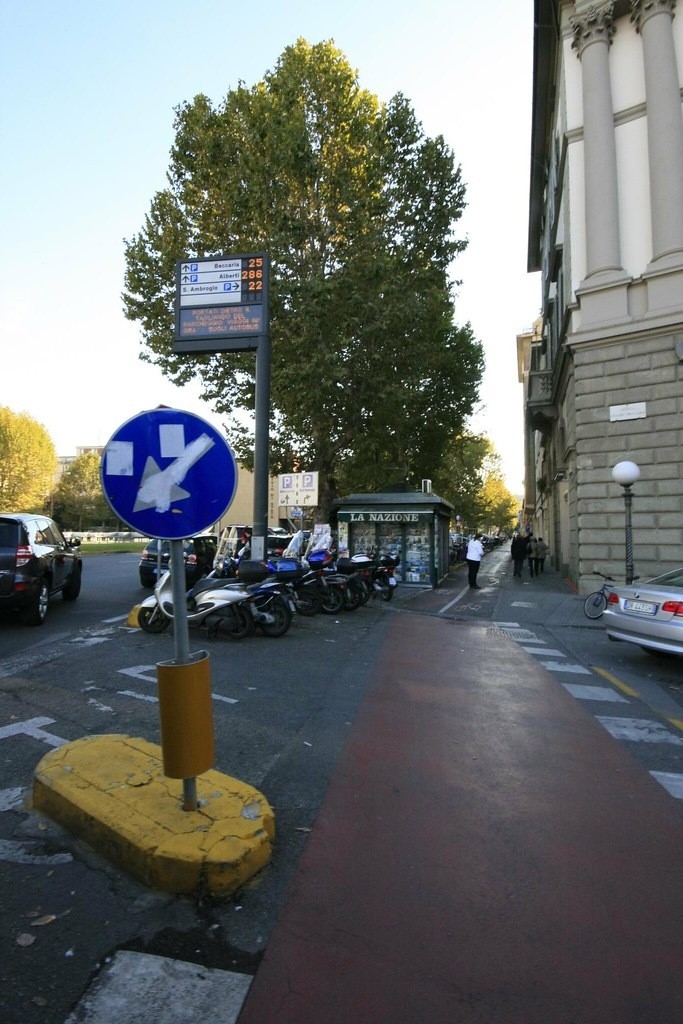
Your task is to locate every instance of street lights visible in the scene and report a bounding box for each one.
[610,460,641,585]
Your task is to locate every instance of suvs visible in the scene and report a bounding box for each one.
[0,512,84,627]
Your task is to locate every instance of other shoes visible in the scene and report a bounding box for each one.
[471,584,481,589]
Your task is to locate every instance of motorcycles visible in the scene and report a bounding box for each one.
[135,530,401,635]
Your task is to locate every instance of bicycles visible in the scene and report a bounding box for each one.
[447,533,508,567]
[583,571,640,620]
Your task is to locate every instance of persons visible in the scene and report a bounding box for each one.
[467,534,484,589]
[510,532,549,578]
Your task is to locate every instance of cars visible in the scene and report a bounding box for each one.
[241,534,295,565]
[221,525,286,556]
[601,566,683,660]
[137,534,233,591]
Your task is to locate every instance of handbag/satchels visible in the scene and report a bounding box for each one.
[527,544,533,555]
[461,544,468,561]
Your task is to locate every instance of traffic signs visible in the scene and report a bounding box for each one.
[173,252,270,352]
